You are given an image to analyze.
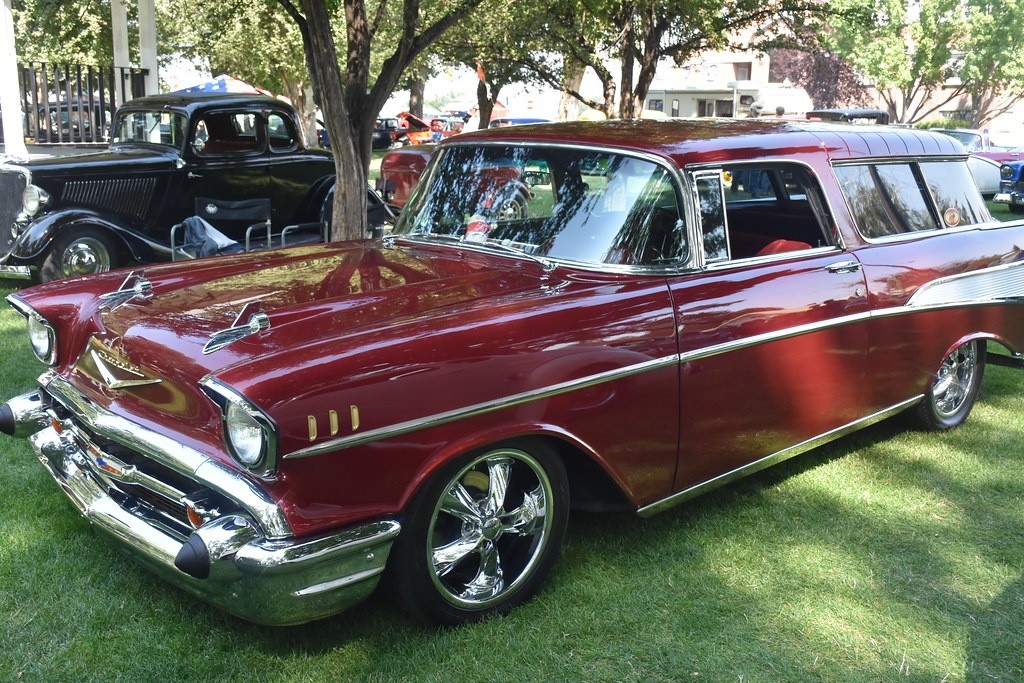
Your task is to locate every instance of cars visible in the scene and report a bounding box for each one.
[0,118,1024,628]
[24,97,1024,228]
[0,94,396,290]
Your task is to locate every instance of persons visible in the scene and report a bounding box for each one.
[774,107,786,119]
[745,102,764,118]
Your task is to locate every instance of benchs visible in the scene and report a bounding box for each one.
[201,139,257,154]
[727,209,828,259]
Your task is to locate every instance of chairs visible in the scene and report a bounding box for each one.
[754,239,814,257]
[171,174,402,263]
[618,205,684,267]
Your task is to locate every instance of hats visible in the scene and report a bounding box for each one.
[750,101,764,118]
